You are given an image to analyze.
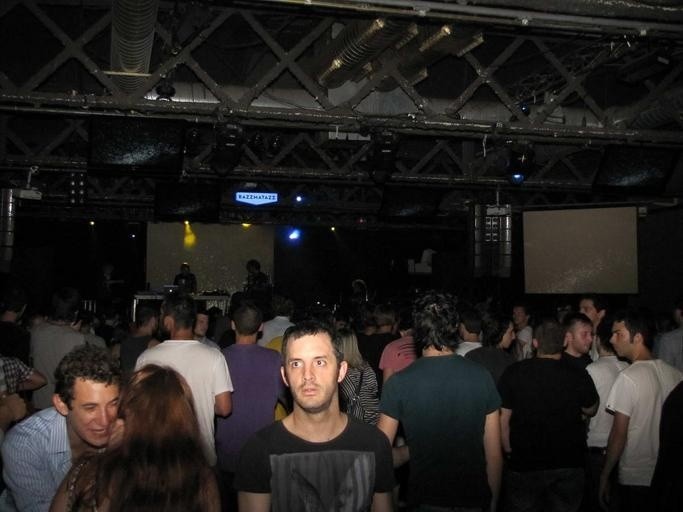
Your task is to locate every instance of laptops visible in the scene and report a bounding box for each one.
[164,285,180,294]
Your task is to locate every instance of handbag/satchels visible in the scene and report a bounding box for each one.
[342,396,357,416]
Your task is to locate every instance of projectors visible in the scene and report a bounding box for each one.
[486,204,512,216]
[12,186,43,202]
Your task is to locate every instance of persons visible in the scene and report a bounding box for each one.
[0,270,683,512]
[245,261,270,295]
[172,263,197,295]
